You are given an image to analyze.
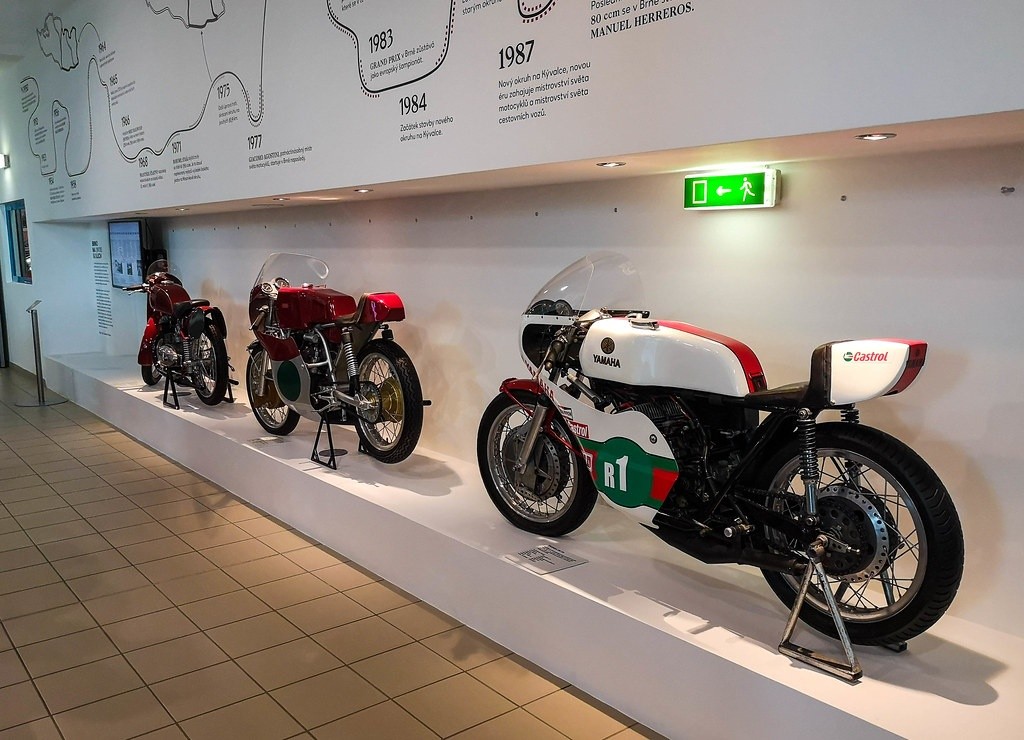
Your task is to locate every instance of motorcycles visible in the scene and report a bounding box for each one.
[245,251,432,471]
[122,259,240,410]
[475,249,967,685]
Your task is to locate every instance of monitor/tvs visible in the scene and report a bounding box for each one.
[108,218,147,290]
